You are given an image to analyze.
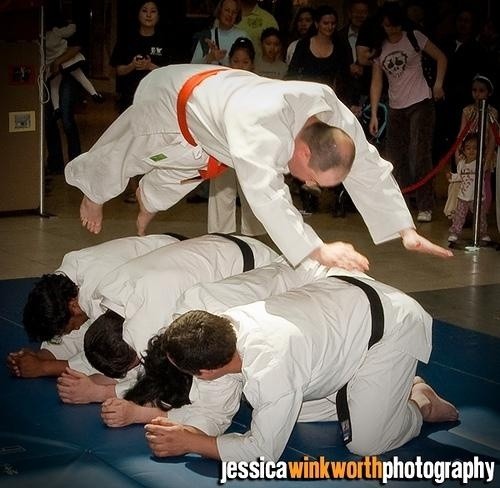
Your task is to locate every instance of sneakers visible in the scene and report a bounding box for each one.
[417,210,432,222]
[448,235,458,242]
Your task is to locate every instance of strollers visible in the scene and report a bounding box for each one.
[302,100,389,216]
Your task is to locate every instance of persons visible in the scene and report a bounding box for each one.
[7,232,190,379]
[56,234,282,404]
[144,274,460,468]
[34,0,499,271]
[99,255,373,429]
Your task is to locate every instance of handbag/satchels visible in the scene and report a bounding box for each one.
[407,29,436,87]
